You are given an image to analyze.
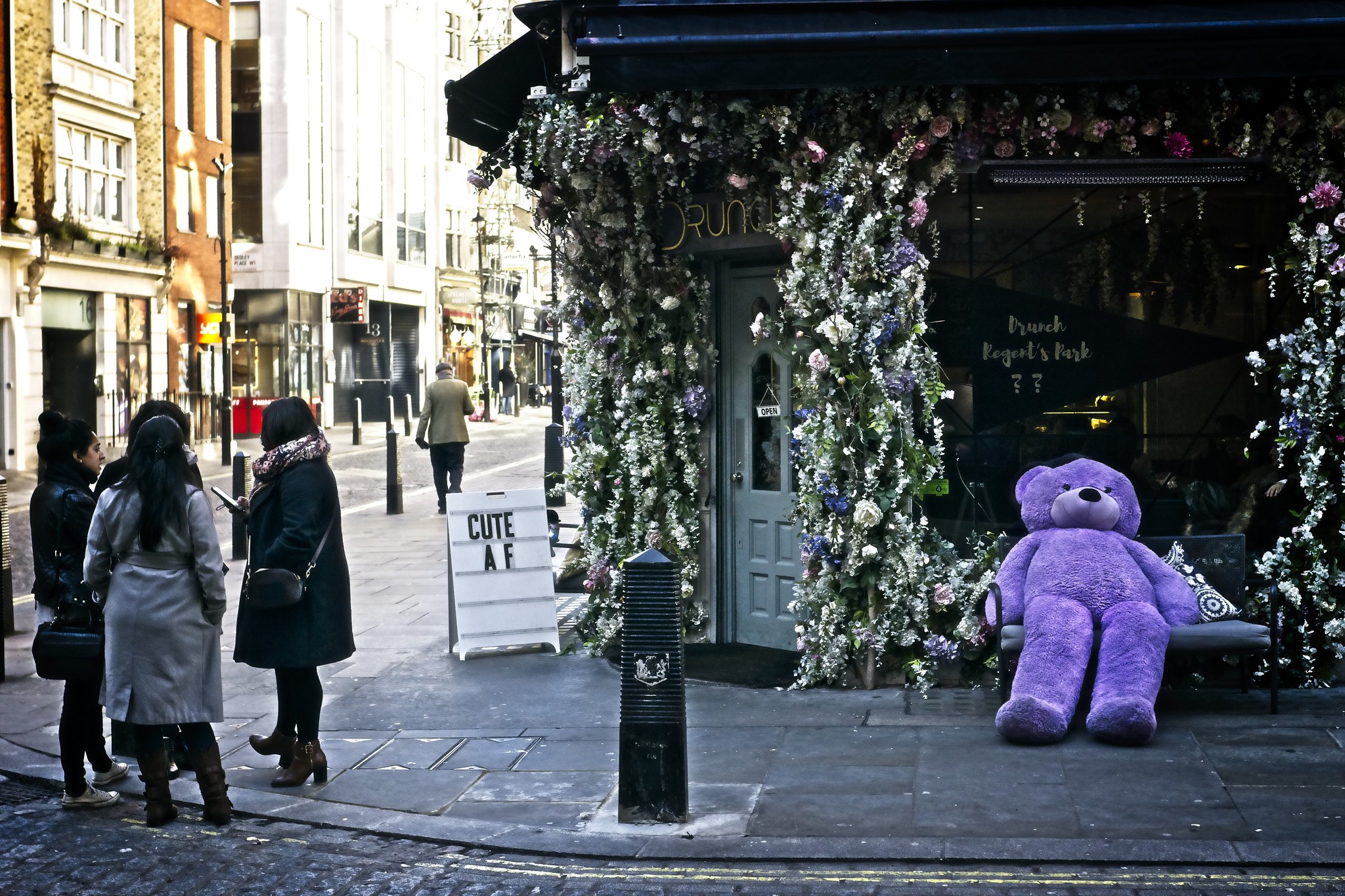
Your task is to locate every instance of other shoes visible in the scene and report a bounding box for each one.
[175,740,196,770]
[438,507,446,514]
[166,755,179,780]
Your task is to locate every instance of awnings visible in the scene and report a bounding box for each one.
[444,30,562,192]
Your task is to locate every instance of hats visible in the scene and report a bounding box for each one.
[435,363,452,372]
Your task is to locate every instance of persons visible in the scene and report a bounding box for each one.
[30,410,129,808]
[499,361,516,415]
[84,414,233,827]
[93,399,203,778]
[232,396,356,788]
[415,363,475,514]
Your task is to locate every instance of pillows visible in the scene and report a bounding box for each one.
[1160,540,1245,624]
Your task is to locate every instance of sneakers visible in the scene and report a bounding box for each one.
[62,785,120,808]
[90,755,129,786]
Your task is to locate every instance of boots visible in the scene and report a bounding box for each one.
[188,745,232,825]
[135,741,177,826]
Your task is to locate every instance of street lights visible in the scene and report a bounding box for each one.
[469,206,495,423]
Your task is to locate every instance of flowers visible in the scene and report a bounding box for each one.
[465,78,1345,698]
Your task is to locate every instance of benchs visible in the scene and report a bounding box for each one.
[988,533,1279,713]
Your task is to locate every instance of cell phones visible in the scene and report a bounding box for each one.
[211,486,244,512]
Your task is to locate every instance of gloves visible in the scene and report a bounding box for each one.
[415,438,430,450]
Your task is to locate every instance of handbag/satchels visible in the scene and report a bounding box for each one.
[244,568,306,609]
[32,620,103,679]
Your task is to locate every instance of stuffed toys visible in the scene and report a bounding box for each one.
[985,458,1200,747]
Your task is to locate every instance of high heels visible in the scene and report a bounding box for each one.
[271,741,328,787]
[248,725,296,768]
